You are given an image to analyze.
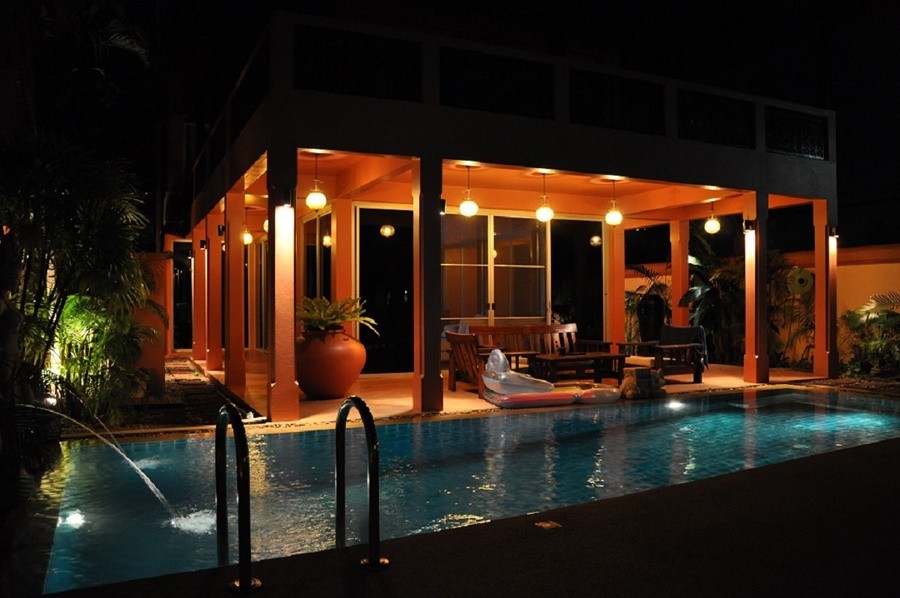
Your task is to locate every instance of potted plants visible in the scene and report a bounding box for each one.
[296,295,380,399]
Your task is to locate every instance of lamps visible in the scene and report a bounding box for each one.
[600,175,627,226]
[299,151,334,211]
[702,198,721,234]
[456,161,481,219]
[529,168,557,223]
[244,207,253,245]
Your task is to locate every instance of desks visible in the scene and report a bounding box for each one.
[522,352,630,384]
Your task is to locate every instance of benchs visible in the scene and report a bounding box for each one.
[468,323,612,356]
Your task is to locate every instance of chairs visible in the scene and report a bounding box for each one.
[443,331,540,398]
[615,325,710,388]
